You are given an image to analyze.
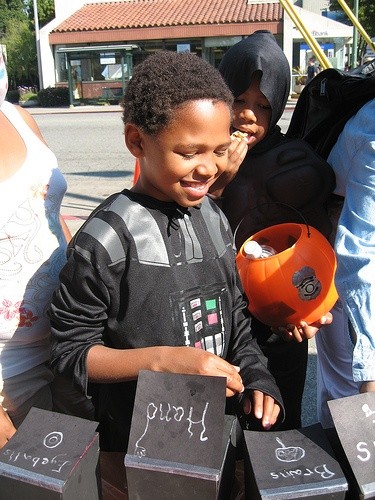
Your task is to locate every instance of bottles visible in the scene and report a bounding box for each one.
[244,240,277,259]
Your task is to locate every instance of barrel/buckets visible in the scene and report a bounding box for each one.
[235,202,338,330]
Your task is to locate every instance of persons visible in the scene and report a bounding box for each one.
[208,30,338,430]
[1,44,95,443]
[318,42,375,429]
[46,51,284,500]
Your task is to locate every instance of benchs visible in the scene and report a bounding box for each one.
[99,87,128,104]
